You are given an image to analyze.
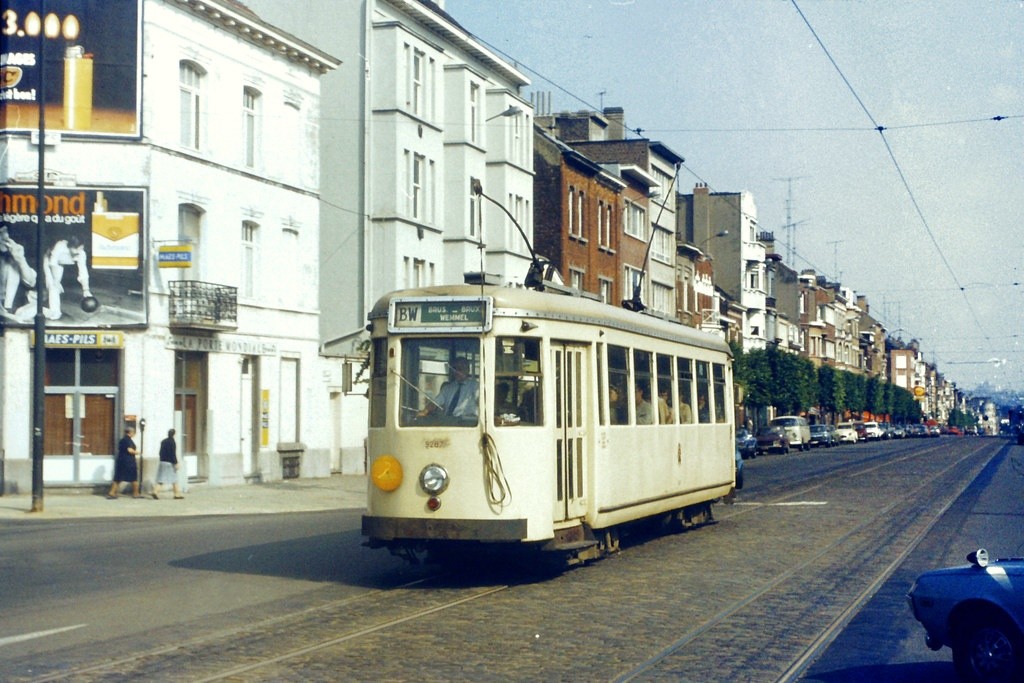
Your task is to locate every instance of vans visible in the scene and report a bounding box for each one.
[770,415,811,452]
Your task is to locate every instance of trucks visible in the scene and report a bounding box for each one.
[1008,405,1024,445]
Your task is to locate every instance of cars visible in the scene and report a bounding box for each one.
[810,424,839,448]
[736,447,744,489]
[837,421,881,444]
[906,546,1024,683]
[756,426,789,456]
[880,423,963,440]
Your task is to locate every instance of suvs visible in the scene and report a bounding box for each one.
[737,428,757,459]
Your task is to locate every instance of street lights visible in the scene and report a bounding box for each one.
[694,229,729,330]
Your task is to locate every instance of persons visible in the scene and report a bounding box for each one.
[0,225,39,325]
[14,228,92,324]
[105,428,145,500]
[149,429,187,500]
[609,374,725,424]
[413,354,479,422]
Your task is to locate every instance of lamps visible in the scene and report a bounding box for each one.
[647,191,662,199]
[486,105,522,122]
[701,230,728,245]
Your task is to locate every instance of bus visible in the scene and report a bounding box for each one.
[362,286,736,567]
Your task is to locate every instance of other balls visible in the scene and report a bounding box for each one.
[80,296,99,313]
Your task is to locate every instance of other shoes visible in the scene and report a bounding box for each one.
[106,495,118,499]
[151,493,158,500]
[174,495,185,500]
[133,495,144,498]
